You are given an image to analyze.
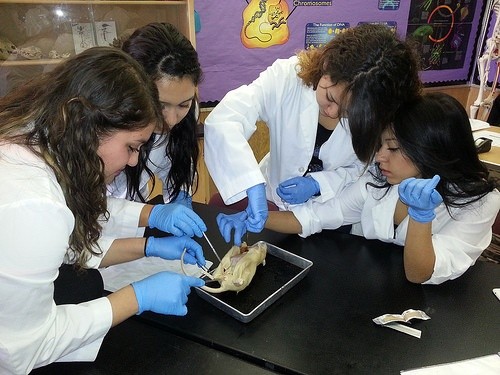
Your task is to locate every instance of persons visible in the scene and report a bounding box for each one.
[216,93,500,286]
[203,21,423,233]
[105,22,207,239]
[0,47,206,375]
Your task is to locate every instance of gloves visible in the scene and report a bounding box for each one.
[240,184,270,234]
[147,202,208,238]
[130,270,205,316]
[397,173,443,222]
[145,235,206,268]
[216,208,249,247]
[276,174,321,205]
[169,189,193,210]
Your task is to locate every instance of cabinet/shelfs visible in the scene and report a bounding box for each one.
[0,0,196,199]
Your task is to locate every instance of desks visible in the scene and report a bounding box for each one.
[29,279,284,375]
[473,126,500,261]
[56,194,500,375]
